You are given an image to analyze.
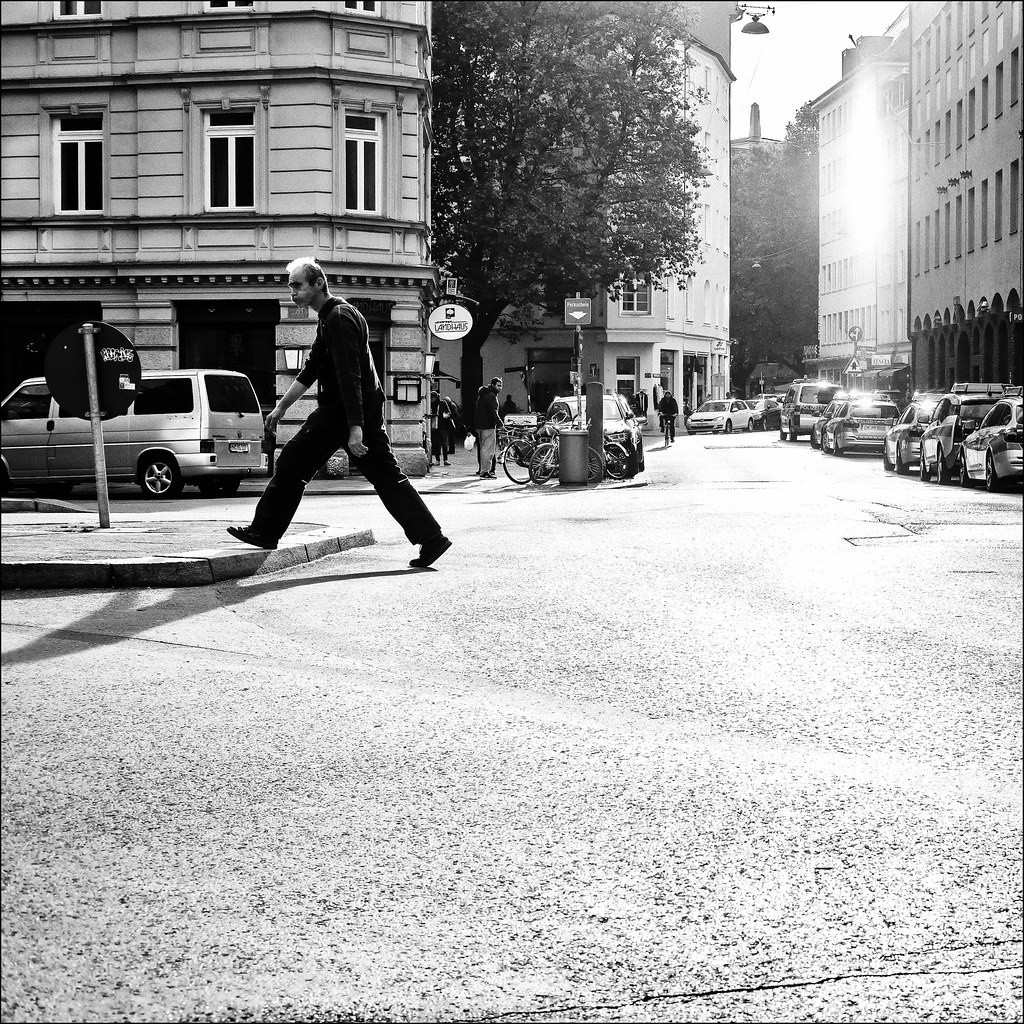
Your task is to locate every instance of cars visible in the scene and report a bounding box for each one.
[744,393,786,431]
[686,397,754,435]
[883,390,949,475]
[914,382,1015,486]
[546,394,649,477]
[956,385,1024,491]
[808,392,902,456]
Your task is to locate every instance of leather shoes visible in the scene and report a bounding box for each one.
[409,533,452,567]
[227,526,279,549]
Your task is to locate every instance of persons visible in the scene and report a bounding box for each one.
[227,256,454,569]
[423,390,460,466]
[466,377,517,480]
[683,391,733,428]
[897,389,913,413]
[657,391,679,443]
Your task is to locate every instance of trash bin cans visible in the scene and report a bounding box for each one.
[557,429,589,486]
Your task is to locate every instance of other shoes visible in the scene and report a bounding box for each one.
[444,461,451,465]
[434,461,440,466]
[480,473,497,479]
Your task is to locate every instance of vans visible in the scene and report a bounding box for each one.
[0,368,269,501]
[776,376,845,441]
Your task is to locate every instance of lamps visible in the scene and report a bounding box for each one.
[741,12,769,35]
[283,345,304,373]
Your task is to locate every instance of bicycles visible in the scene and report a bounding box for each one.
[496,408,630,485]
[658,412,678,447]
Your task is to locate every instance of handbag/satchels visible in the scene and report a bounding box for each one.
[438,418,455,436]
[464,435,476,450]
